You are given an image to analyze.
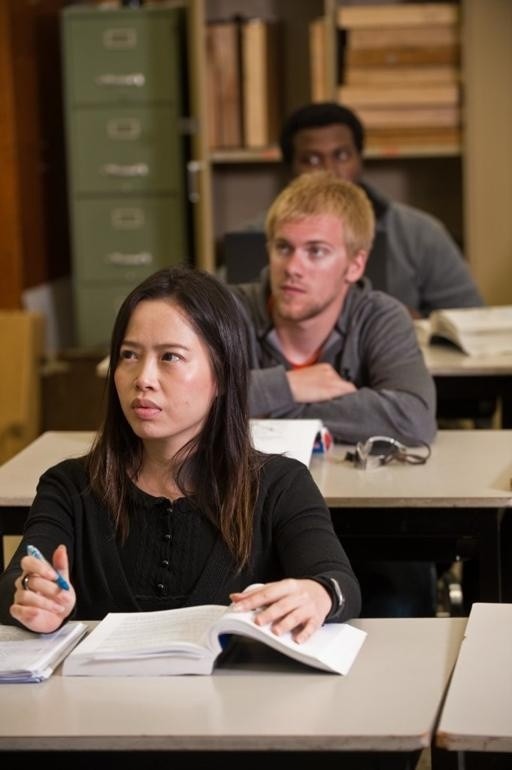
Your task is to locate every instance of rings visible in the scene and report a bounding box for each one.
[21,574,30,590]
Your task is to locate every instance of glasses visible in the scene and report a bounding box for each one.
[353,435,431,470]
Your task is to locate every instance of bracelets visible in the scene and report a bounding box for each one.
[343,434,432,472]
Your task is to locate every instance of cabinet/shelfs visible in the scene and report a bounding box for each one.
[192,0,469,277]
[62,0,187,350]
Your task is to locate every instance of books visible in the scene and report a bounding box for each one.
[0,617,92,686]
[57,602,371,681]
[246,414,334,469]
[204,17,326,149]
[333,1,464,157]
[411,304,511,360]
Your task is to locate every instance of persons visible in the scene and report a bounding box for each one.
[0,258,366,646]
[226,169,440,455]
[228,97,486,323]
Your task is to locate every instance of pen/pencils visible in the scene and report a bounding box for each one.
[27,545,73,593]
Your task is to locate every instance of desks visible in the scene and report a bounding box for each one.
[0,614,469,769]
[436,600,510,769]
[94,343,511,426]
[0,429,512,604]
